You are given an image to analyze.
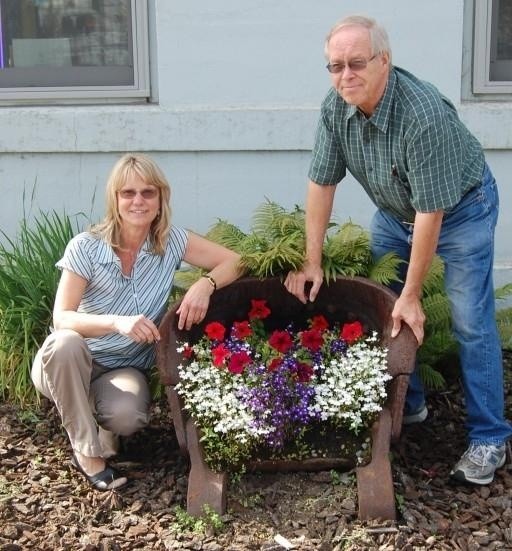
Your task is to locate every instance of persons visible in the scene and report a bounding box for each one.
[27,151,248,496]
[282,15,508,492]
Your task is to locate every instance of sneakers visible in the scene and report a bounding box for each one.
[400,406,429,427]
[448,442,506,487]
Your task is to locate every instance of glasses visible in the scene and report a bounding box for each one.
[326,54,378,74]
[118,188,159,200]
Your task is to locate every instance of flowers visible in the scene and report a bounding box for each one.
[175,297,398,483]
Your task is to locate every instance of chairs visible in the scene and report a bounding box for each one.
[151,271,419,525]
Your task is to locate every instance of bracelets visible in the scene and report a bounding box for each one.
[200,275,216,293]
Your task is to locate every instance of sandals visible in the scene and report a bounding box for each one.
[68,454,132,492]
